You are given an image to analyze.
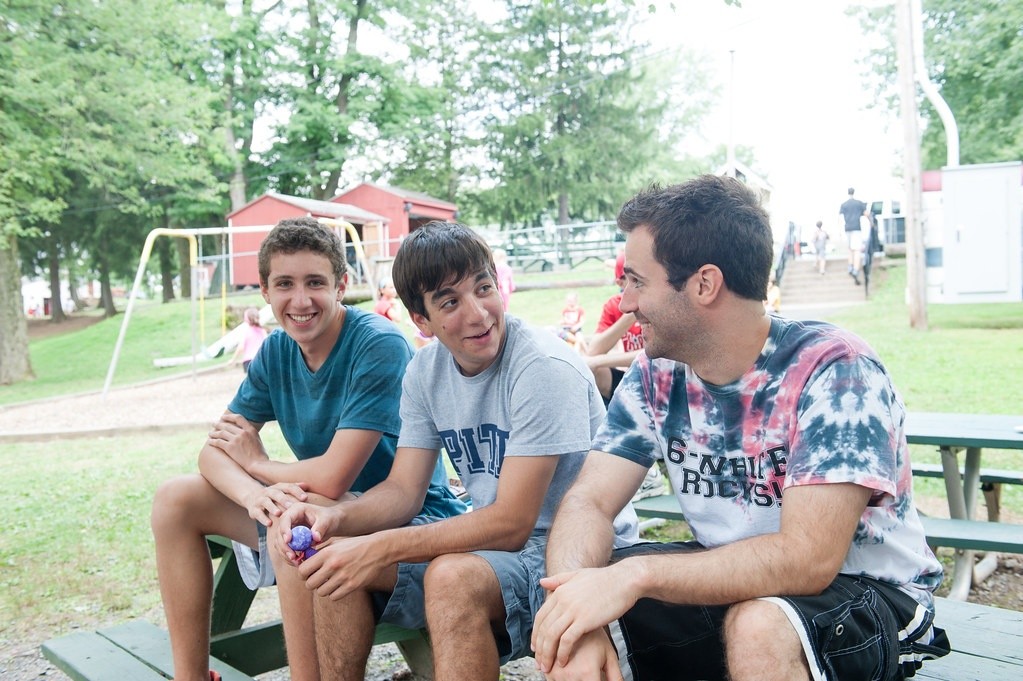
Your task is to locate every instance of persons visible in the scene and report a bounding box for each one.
[229,309,269,374]
[273,220,638,681]
[813,221,830,274]
[762,270,782,317]
[490,249,515,313]
[374,276,402,321]
[150,216,467,681]
[528,175,944,681]
[557,291,583,347]
[839,187,874,285]
[587,248,646,402]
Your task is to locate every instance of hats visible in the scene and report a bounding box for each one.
[615,255,625,279]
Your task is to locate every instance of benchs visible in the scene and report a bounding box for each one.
[630,462,1023,602]
[40,620,256,681]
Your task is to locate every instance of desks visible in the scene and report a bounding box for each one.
[204,530,1023,681]
[901,411,1023,602]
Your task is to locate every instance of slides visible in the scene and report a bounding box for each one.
[203,304,276,358]
